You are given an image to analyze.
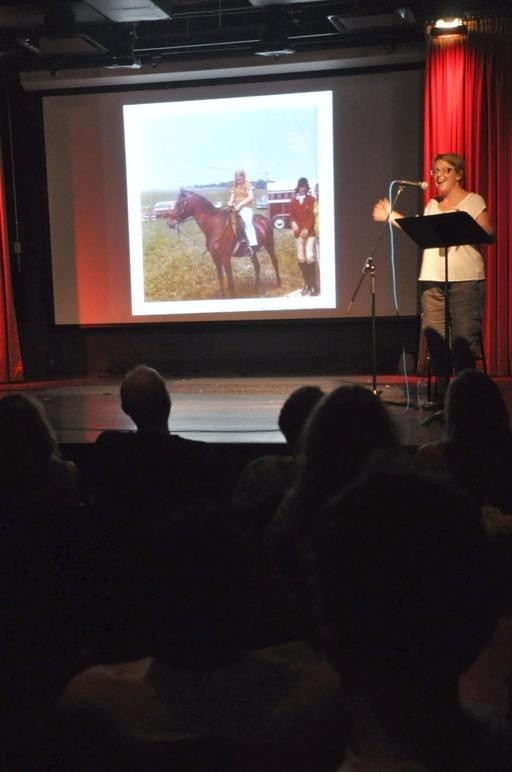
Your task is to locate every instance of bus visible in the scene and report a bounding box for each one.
[265,178,321,231]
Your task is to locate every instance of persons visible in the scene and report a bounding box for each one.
[371,151,497,398]
[288,177,321,297]
[2,365,511,770]
[227,169,259,253]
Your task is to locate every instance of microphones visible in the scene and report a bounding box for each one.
[401,180,428,190]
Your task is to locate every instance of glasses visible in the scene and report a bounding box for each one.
[430,166,457,174]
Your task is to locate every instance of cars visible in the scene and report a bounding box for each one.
[154,199,175,218]
[254,195,269,210]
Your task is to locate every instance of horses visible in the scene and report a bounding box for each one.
[164,190,283,298]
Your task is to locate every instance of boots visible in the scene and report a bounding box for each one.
[298,260,317,297]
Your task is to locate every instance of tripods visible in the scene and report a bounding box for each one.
[369,265,417,409]
[420,247,451,425]
